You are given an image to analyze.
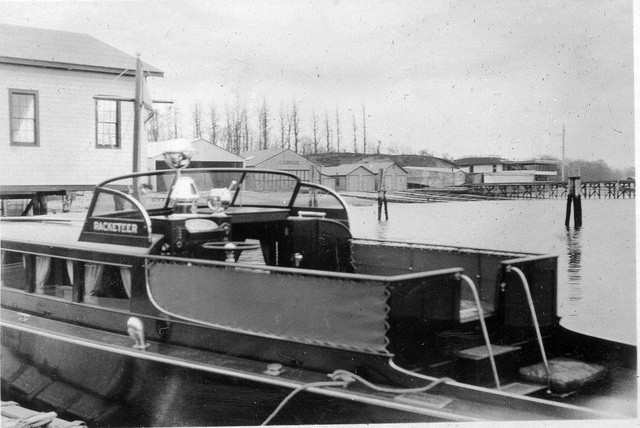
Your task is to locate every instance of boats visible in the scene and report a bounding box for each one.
[1,168,635,421]
